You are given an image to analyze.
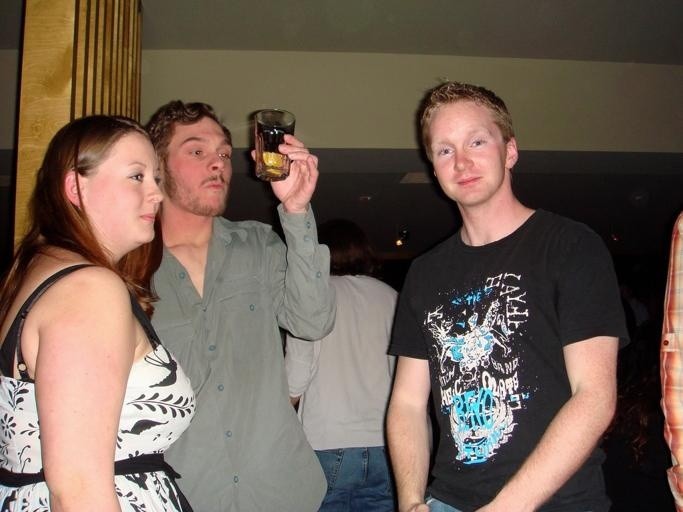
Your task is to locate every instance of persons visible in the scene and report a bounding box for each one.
[285,219,400,512]
[144,100,337,512]
[0,115,195,512]
[385,78,631,512]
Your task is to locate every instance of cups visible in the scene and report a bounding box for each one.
[250,106,296,182]
